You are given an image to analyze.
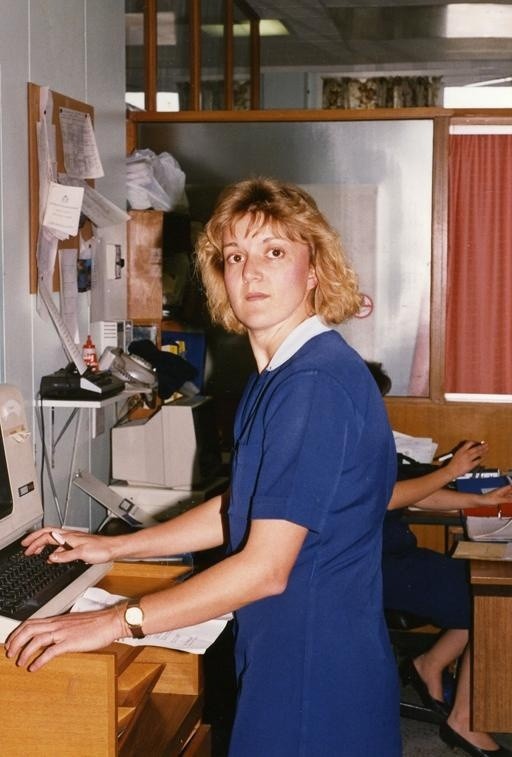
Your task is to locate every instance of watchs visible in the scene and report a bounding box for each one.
[124,593,146,640]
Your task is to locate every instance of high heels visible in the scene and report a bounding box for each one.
[439,716,512,757]
[401,659,451,718]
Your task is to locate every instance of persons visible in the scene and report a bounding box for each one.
[360,358,512,757]
[4,177,402,757]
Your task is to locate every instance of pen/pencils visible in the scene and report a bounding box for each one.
[434,439,489,464]
[47,530,90,566]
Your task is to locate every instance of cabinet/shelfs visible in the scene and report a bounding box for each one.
[1,555,212,757]
[32,390,132,409]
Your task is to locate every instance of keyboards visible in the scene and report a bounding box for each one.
[0,535,118,629]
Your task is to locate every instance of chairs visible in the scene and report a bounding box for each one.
[383,610,446,725]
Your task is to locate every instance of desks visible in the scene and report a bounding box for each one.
[406,511,512,734]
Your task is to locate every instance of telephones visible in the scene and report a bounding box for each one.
[97,345,157,391]
